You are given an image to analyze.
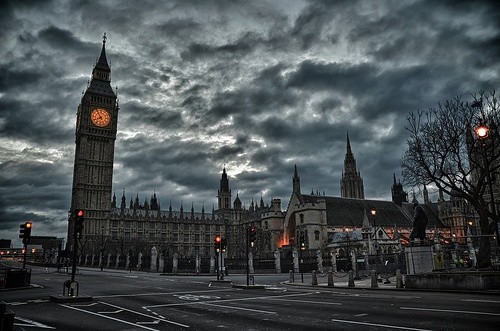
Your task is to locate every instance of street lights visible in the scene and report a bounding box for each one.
[370,209,381,281]
[101,225,105,271]
[223,217,229,239]
[467,216,476,235]
[474,124,500,247]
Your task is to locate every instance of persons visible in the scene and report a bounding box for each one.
[407,200,428,241]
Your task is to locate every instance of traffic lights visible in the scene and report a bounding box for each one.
[296,234,305,251]
[18,221,32,239]
[221,237,228,253]
[213,233,220,246]
[73,209,85,239]
[247,226,257,242]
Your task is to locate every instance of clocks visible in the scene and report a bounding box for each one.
[91,109,110,128]
